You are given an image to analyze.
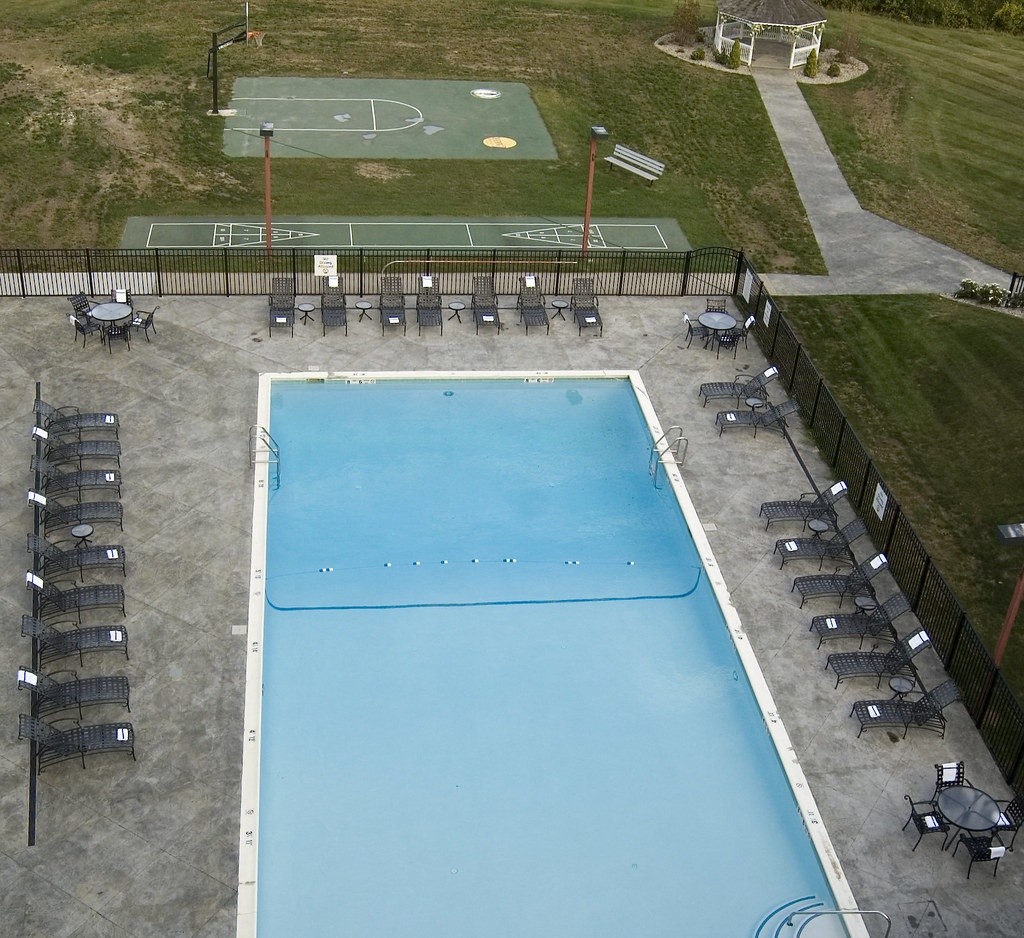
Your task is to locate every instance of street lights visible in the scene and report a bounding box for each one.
[986,523,1024,675]
[259,120,277,258]
[581,126,612,261]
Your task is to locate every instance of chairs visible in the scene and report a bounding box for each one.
[759,479,962,741]
[416,276,442,336]
[683,299,755,360]
[699,366,801,441]
[18,383,138,848]
[322,275,348,337]
[517,276,550,336]
[902,760,1024,879]
[570,278,603,336]
[380,276,407,336]
[66,288,160,355]
[471,276,501,336]
[268,276,296,338]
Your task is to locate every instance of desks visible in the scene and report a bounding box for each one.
[697,311,737,350]
[447,302,466,323]
[852,595,876,616]
[808,519,829,540]
[551,300,568,321]
[355,301,373,322]
[298,303,315,325]
[888,677,914,701]
[91,302,134,345]
[937,785,1001,851]
[745,396,765,412]
